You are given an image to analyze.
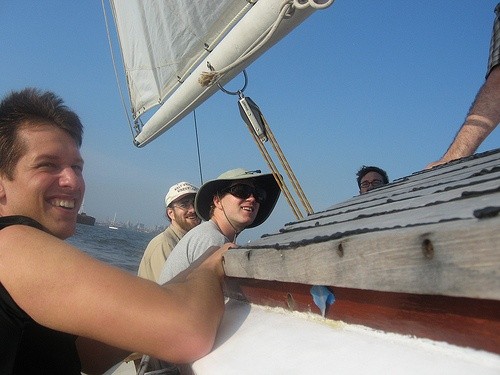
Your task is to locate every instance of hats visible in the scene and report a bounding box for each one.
[165,180,199,210]
[194,168,284,230]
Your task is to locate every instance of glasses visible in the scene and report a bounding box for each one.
[224,183,270,203]
[358,180,385,190]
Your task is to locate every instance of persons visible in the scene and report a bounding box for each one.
[423,2,500,169]
[0,85,239,375]
[158,169,284,286]
[137,182,202,285]
[355,165,390,194]
